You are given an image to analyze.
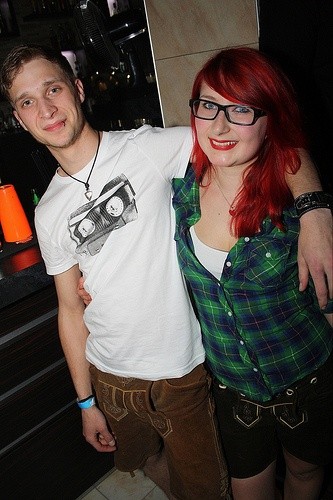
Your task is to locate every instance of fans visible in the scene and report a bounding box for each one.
[73,0,147,88]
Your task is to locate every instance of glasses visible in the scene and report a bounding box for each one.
[188,98,269,126]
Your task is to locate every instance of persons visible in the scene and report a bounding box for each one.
[76,46,333,500]
[0,45,333,500]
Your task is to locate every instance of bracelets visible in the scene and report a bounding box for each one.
[77,395,95,409]
[293,190,333,219]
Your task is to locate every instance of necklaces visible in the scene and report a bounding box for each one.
[59,130,100,200]
[212,175,237,210]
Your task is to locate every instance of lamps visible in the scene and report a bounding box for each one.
[0,184,33,244]
